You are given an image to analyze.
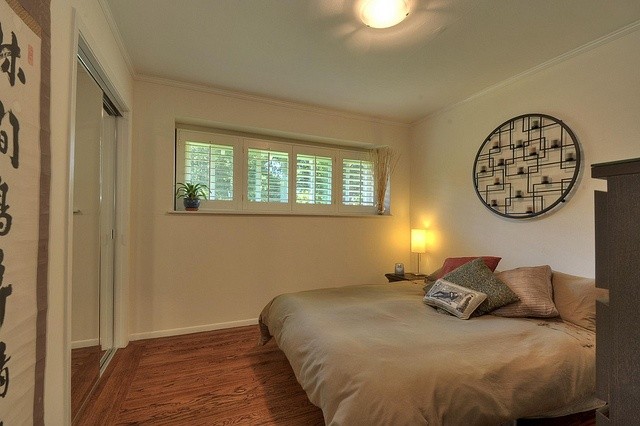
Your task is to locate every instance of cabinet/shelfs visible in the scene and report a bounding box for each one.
[592,159,639,426]
[472,114,581,220]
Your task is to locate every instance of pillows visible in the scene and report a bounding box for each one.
[422,279,487,320]
[551,270,609,332]
[423,259,519,318]
[437,256,502,286]
[488,265,560,317]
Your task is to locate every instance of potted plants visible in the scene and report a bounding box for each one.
[175,182,211,211]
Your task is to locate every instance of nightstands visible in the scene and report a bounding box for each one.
[385,273,428,282]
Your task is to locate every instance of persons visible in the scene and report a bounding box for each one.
[431,290,463,301]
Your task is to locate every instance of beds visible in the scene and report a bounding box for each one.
[259,279,607,425]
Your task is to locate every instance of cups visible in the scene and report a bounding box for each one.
[395,263,404,275]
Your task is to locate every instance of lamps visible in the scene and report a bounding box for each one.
[411,230,427,276]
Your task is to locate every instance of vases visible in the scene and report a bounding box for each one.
[373,202,386,215]
